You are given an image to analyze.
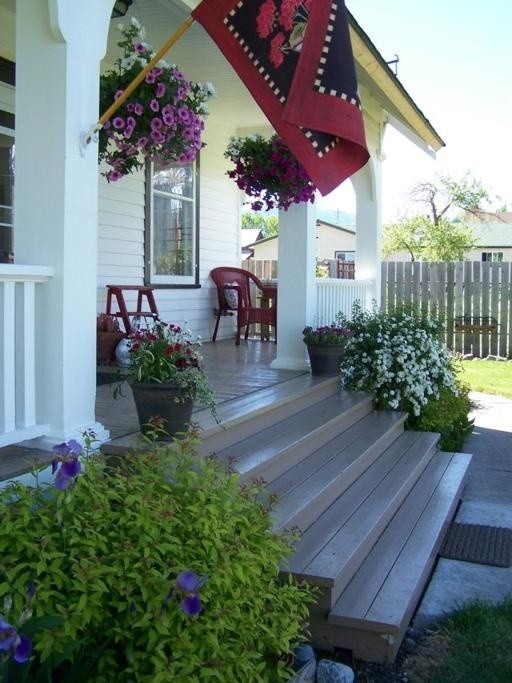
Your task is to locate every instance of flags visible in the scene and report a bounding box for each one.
[191,0,370,195]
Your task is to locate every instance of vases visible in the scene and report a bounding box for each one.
[128,379,197,441]
[97,332,122,366]
[114,337,134,366]
[305,342,344,377]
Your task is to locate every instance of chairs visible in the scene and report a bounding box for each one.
[210,266,277,346]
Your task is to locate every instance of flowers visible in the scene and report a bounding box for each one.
[108,318,230,434]
[97,18,217,183]
[225,133,317,214]
[97,313,120,332]
[302,323,356,345]
